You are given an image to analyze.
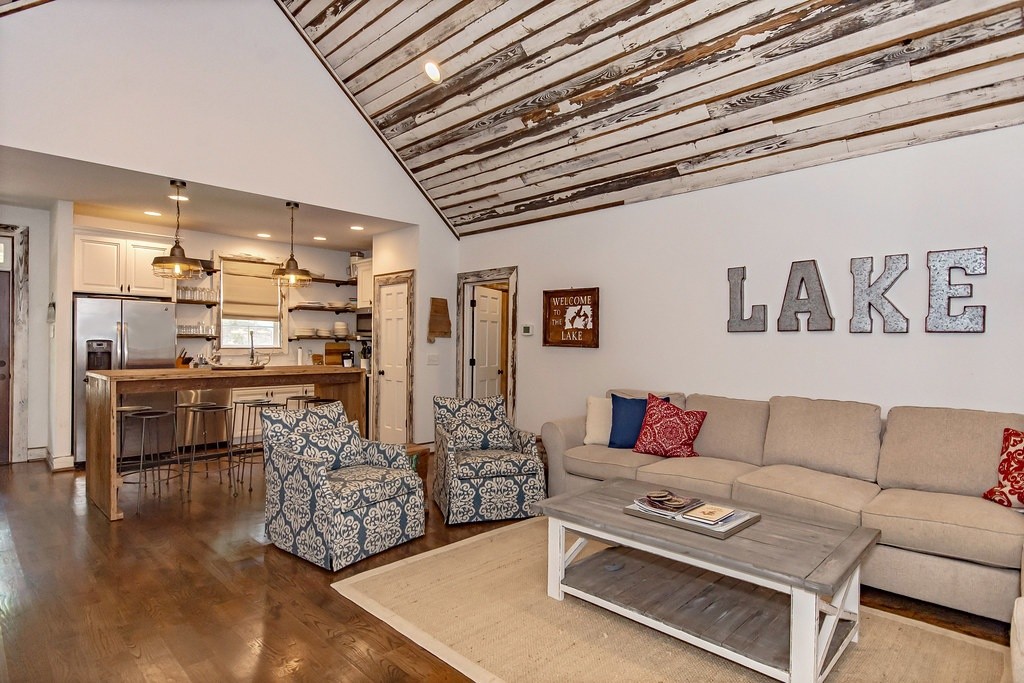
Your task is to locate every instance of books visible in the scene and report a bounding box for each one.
[681,501,735,525]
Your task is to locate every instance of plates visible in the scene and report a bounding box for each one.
[333,322,348,337]
[294,327,315,337]
[317,329,331,338]
[296,301,326,306]
[327,306,345,308]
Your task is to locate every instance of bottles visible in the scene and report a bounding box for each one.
[348,251,364,280]
[343,354,351,367]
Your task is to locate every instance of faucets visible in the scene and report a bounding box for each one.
[249,331,254,366]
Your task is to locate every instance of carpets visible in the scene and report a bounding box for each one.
[330,516,1014,683]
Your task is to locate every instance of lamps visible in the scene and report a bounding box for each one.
[271,200,313,288]
[151,179,205,280]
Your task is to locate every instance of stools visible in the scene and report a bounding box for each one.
[116,396,337,517]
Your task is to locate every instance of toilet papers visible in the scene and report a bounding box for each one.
[298,349,303,365]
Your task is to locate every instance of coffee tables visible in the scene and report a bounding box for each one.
[398,442,431,514]
[529,475,883,683]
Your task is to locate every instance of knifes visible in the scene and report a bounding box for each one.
[182,357,193,364]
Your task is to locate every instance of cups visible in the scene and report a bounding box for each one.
[360,360,370,369]
[177,321,216,335]
[177,285,216,302]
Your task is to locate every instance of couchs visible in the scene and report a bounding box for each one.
[259,400,424,571]
[542,387,1024,624]
[432,395,548,526]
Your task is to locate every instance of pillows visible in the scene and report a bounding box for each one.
[446,416,514,451]
[981,427,1024,509]
[608,393,671,449]
[285,420,366,472]
[632,394,707,458]
[582,396,612,445]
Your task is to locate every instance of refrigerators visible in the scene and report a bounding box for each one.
[73,295,175,468]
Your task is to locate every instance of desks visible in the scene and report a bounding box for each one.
[85,364,366,522]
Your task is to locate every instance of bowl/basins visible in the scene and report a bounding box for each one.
[328,302,345,306]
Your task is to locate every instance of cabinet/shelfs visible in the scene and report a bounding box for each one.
[74,234,177,302]
[351,259,374,308]
[177,270,222,340]
[225,384,314,446]
[288,278,355,339]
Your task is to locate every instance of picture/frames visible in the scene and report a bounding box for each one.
[543,285,600,348]
